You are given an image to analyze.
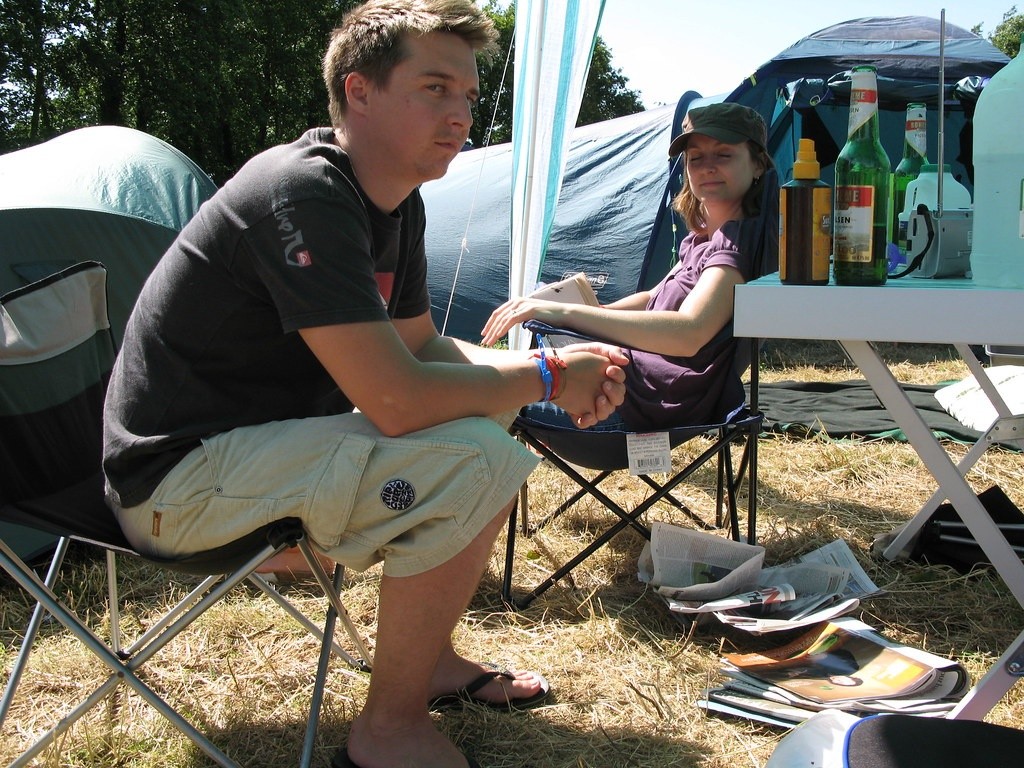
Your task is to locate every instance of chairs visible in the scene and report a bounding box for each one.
[0,208,375,768]
[502,157,779,611]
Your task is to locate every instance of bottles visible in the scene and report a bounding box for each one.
[900,162,971,217]
[777,137,833,286]
[833,65,892,286]
[970,31,1024,288]
[887,102,931,264]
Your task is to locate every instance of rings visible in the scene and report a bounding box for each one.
[512,311,517,315]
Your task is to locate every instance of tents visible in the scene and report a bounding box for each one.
[417,17,1012,348]
[0,125,218,571]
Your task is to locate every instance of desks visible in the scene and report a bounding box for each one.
[732,254,1024,724]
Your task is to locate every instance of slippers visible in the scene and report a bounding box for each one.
[428,662,551,712]
[332,749,482,768]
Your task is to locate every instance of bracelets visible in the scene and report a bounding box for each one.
[527,333,568,402]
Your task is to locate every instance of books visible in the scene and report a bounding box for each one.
[527,272,600,307]
[695,615,974,731]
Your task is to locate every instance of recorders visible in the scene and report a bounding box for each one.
[908,9,976,280]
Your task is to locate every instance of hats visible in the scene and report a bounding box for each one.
[668,103,768,157]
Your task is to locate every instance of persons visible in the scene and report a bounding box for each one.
[481,103,775,470]
[101,0,629,768]
